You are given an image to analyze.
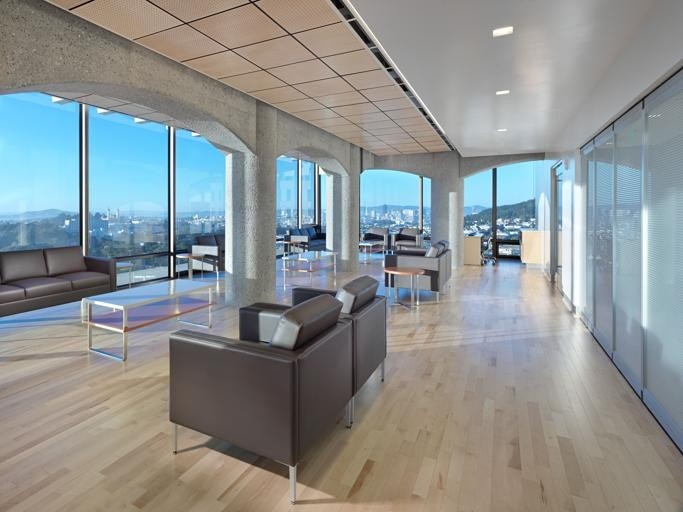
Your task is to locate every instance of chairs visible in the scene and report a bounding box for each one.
[359,226,451,303]
[252,275,387,425]
[168,295,355,506]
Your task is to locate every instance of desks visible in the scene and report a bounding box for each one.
[80,279,217,361]
[175,252,205,281]
[383,266,425,313]
[281,249,339,284]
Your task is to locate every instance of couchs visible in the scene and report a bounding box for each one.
[284,223,326,254]
[188,233,225,279]
[0,244,117,318]
